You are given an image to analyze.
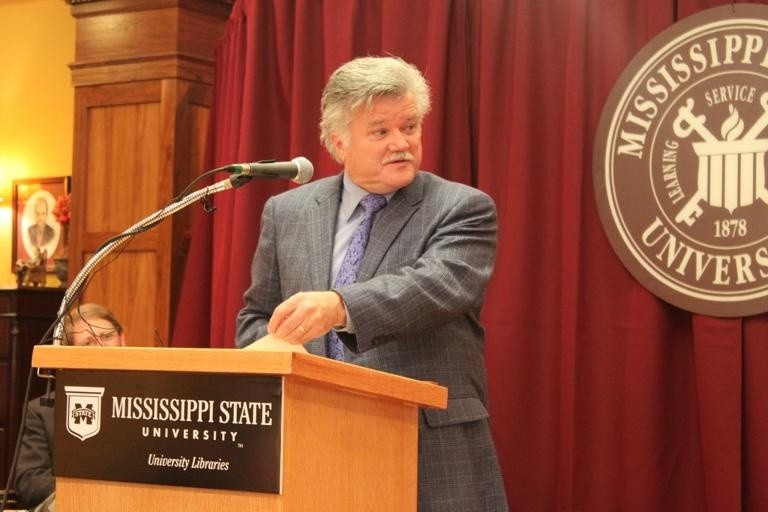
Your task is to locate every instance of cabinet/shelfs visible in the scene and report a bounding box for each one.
[0,286,64,486]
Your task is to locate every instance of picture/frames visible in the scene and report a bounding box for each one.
[12,175,70,273]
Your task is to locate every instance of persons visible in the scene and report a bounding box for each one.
[12,298,127,511]
[231,50,511,512]
[26,196,56,249]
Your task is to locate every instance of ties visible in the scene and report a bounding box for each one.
[329,192,388,362]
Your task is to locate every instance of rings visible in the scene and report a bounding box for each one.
[297,326,307,336]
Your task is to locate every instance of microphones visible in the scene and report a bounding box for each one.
[223,156,314,184]
